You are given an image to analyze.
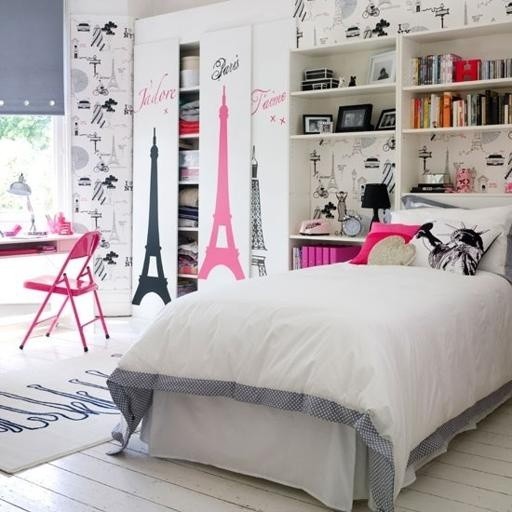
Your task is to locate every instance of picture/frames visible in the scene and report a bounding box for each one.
[375,108,396,132]
[303,114,332,133]
[367,49,395,83]
[335,103,373,132]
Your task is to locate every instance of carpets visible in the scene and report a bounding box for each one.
[0,338,141,475]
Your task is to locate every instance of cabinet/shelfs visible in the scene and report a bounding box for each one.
[399,22,510,210]
[133,20,291,318]
[289,33,398,271]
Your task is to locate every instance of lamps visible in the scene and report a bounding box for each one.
[8,174,48,235]
[361,183,390,235]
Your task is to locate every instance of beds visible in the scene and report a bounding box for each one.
[106,261,512,512]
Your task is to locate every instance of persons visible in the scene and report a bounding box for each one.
[335,191,349,222]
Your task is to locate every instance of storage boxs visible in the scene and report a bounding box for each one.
[300,78,338,91]
[304,68,333,81]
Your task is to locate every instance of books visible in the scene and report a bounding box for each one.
[408,52,512,130]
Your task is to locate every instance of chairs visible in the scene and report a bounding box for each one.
[19,230,110,352]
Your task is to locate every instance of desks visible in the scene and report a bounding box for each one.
[0,233,95,331]
